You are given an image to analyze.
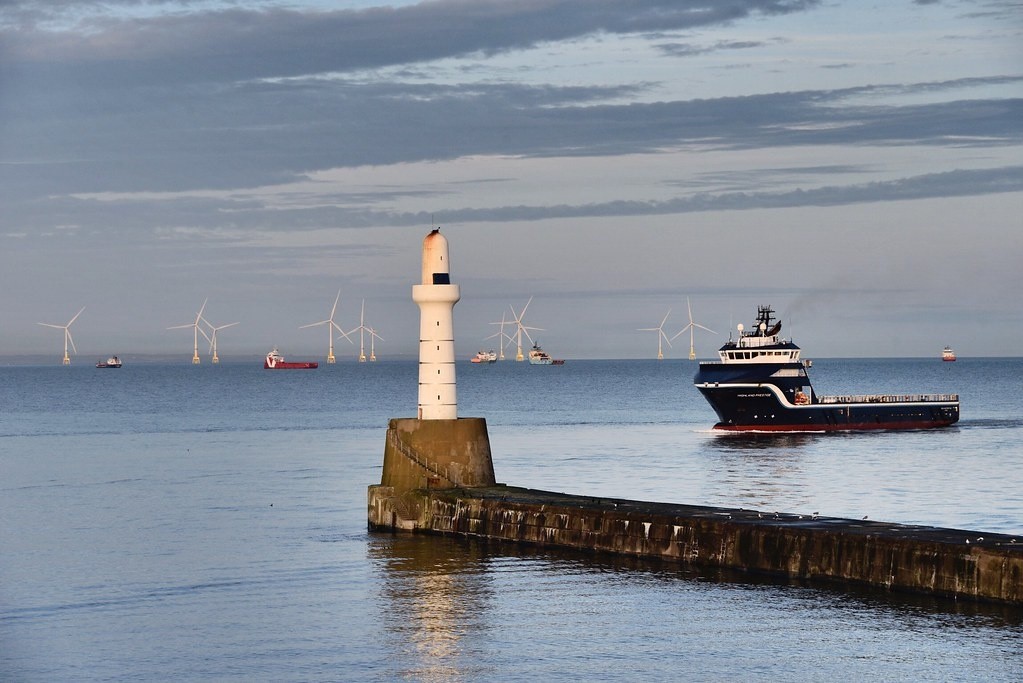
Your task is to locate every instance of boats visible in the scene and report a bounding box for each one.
[470,349,497,363]
[95,355,122,369]
[941,344,956,361]
[528,345,565,365]
[693,303,959,432]
[264,347,319,369]
